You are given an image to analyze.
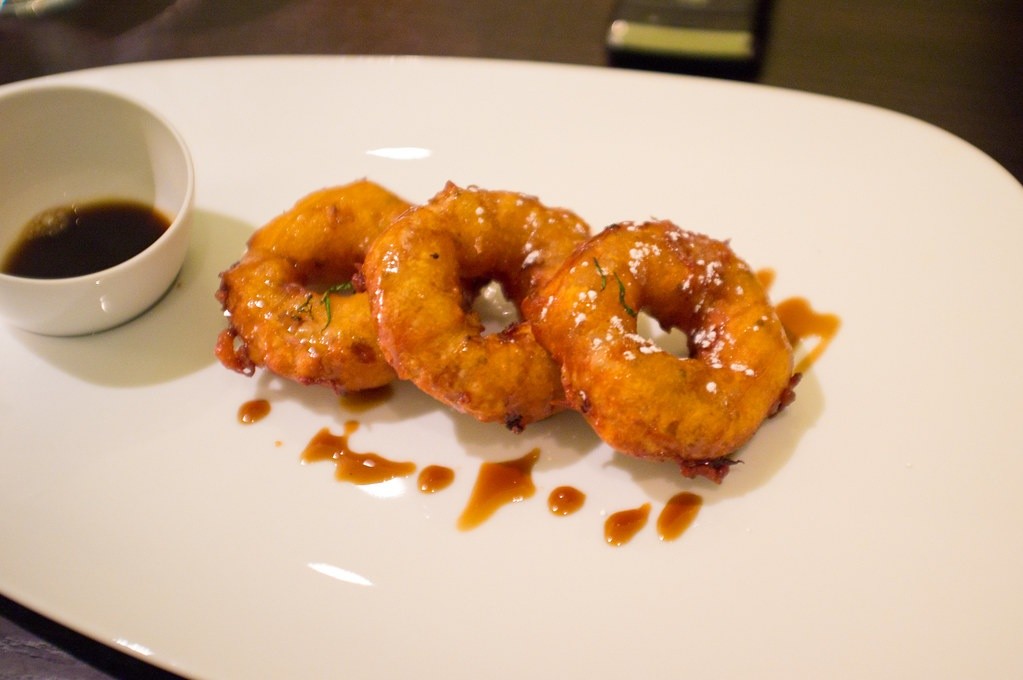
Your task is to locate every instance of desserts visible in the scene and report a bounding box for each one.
[211,178,794,483]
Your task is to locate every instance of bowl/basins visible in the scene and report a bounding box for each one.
[0,82,192,338]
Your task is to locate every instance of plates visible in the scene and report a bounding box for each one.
[0,53,1023,680]
